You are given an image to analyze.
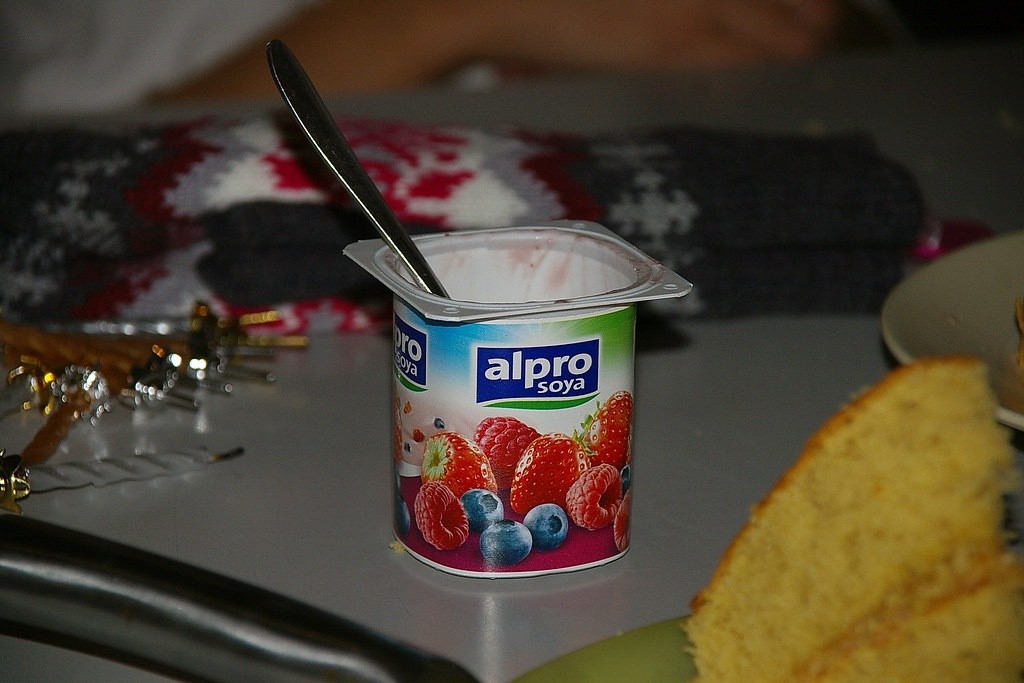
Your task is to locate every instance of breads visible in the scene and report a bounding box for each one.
[677,353,1024,683]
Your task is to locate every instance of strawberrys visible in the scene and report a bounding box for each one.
[421,390,634,515]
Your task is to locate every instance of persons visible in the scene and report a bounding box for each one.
[136,0,838,108]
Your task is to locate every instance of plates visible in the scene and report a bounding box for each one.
[881,234,1024,430]
